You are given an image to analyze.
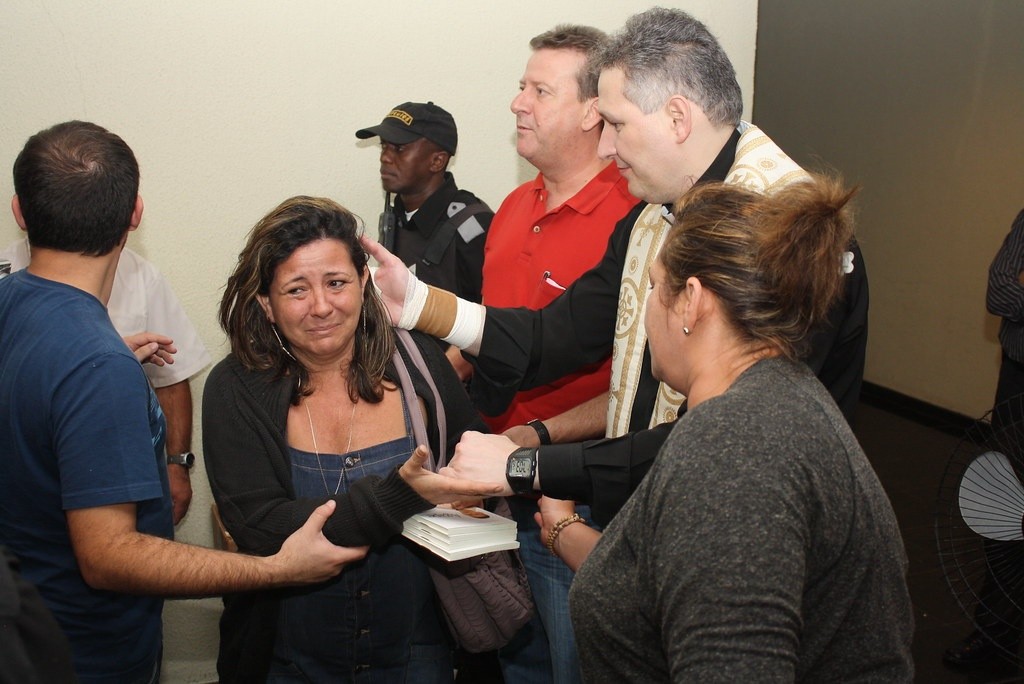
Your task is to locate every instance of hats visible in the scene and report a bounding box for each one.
[355,101,458,156]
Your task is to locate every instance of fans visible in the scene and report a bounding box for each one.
[933,393,1024,670]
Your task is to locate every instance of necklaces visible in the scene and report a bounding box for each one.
[300,392,357,499]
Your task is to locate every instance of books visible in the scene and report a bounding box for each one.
[401,506,521,561]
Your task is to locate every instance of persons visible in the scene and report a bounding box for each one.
[943,208,1024,666]
[356,6,869,531]
[447,21,645,684]
[0,235,213,525]
[0,120,370,684]
[535,181,917,684]
[202,196,497,684]
[438,503,490,519]
[354,101,494,405]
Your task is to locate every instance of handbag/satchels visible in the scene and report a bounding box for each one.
[391,328,534,654]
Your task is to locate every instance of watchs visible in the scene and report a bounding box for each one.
[166,452,195,469]
[506,447,541,497]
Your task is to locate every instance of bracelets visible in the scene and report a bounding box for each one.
[546,515,587,558]
[527,417,553,447]
